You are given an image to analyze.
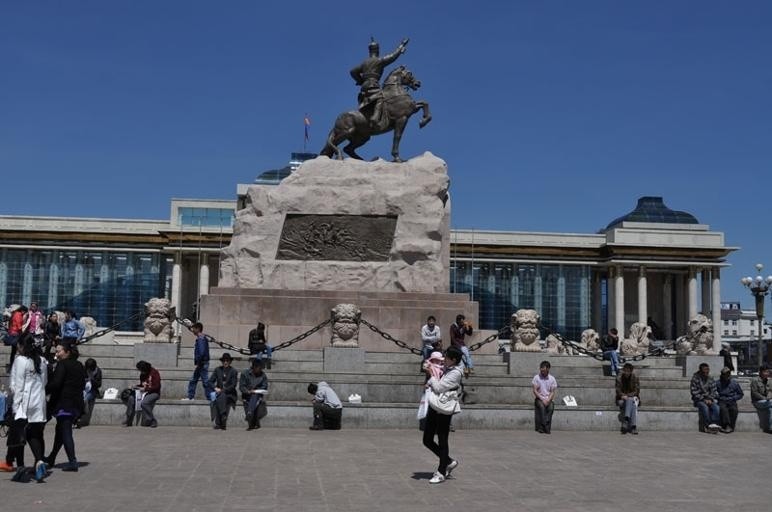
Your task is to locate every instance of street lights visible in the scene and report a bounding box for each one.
[741,263,772,372]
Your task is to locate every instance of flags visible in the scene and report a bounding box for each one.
[305,118,311,140]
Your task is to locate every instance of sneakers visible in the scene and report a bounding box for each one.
[705,424,733,434]
[446,460,458,477]
[429,470,445,483]
[0,462,15,471]
[62,461,79,472]
[621,420,630,433]
[631,429,639,435]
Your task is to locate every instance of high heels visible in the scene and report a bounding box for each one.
[36,461,47,480]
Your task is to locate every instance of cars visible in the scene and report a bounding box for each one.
[737,368,772,378]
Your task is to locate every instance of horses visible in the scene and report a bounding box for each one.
[318,67,433,162]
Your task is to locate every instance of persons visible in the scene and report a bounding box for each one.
[239,358,269,428]
[180,322,214,401]
[0,301,101,483]
[600,328,623,376]
[648,316,659,340]
[307,381,342,430]
[249,322,272,369]
[615,363,641,435]
[532,361,558,434]
[689,342,772,434]
[349,37,411,128]
[416,314,473,484]
[122,360,161,427]
[206,353,238,430]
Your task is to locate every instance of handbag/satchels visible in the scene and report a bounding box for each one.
[3,335,19,344]
[427,388,457,415]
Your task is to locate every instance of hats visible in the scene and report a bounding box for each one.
[720,367,730,374]
[428,352,446,361]
[219,353,234,361]
